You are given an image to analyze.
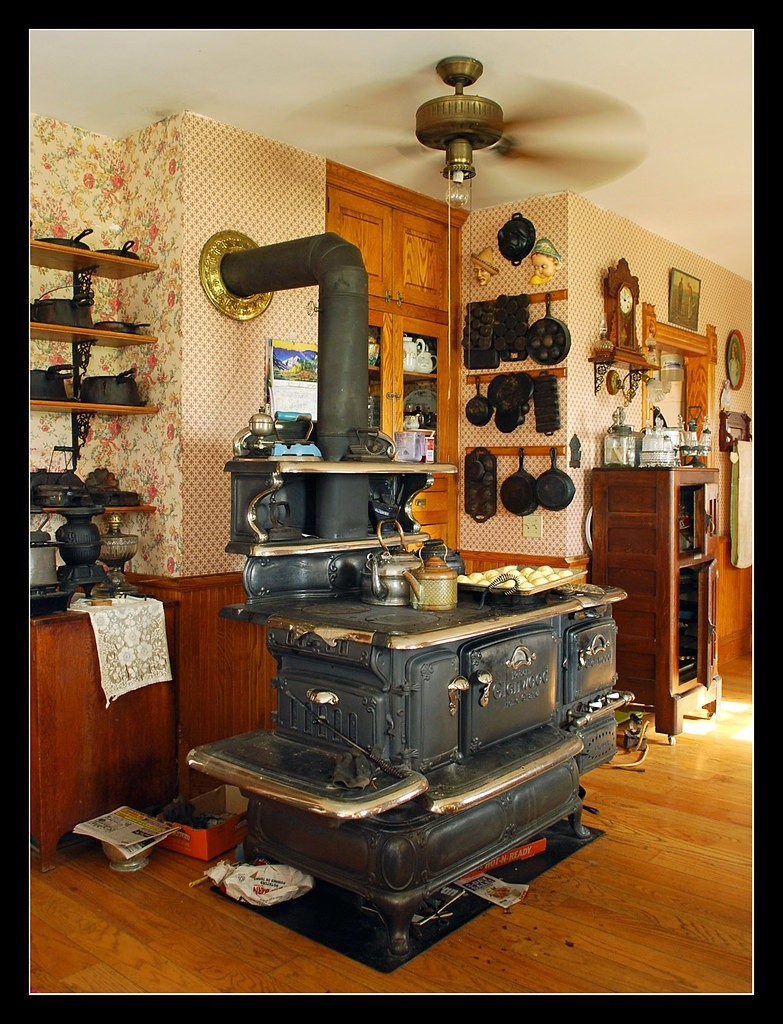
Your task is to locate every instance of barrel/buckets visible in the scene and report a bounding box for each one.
[32,284,93,328]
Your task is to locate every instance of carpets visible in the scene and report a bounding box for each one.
[207,819,606,974]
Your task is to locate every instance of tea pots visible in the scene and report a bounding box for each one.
[402,335,425,372]
[416,346,437,373]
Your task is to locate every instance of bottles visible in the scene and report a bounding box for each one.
[603,426,636,467]
[367,332,379,366]
[641,418,712,465]
[593,333,613,357]
[403,415,419,430]
[411,406,426,428]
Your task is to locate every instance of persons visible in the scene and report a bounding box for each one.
[728,337,740,384]
[677,277,693,320]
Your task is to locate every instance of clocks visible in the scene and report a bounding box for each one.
[588,258,660,371]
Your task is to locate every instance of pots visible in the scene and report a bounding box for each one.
[95,240,140,260]
[35,228,93,251]
[465,375,492,426]
[77,367,139,405]
[536,447,575,511]
[526,293,571,365]
[94,321,150,333]
[500,447,538,517]
[30,363,72,400]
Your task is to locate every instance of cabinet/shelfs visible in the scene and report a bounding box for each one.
[29,239,180,873]
[326,158,470,553]
[591,467,722,746]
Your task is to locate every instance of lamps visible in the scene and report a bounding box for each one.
[415,56,503,210]
[606,370,671,402]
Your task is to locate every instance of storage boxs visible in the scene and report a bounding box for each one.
[154,783,248,861]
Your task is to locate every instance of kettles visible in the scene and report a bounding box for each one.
[360,517,422,606]
[402,539,458,611]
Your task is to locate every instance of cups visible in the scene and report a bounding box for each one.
[394,431,426,462]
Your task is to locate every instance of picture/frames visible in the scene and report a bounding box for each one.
[669,267,701,331]
[726,329,746,390]
